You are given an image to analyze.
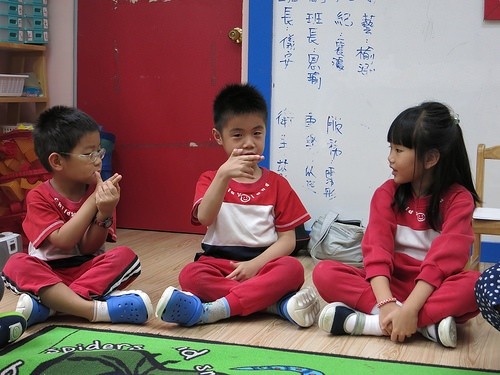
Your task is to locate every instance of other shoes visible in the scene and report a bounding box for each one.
[421,315,457,348]
[318,302,365,336]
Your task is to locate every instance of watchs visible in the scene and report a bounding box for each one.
[92,217,114,229]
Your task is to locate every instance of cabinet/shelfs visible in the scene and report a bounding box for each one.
[0,42,51,126]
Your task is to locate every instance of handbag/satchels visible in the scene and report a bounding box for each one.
[308,213,368,269]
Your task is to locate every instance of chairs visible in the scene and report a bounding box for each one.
[470,144,500,272]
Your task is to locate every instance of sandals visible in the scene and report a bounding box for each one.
[15,293,50,329]
[98,289,154,324]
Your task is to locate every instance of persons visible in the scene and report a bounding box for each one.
[312,102,483,347]
[474,263,500,331]
[0,275,27,348]
[156,83,320,327]
[0,105,153,328]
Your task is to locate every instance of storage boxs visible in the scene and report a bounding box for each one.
[0,1,48,44]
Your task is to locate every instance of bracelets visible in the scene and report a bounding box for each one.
[377,298,402,308]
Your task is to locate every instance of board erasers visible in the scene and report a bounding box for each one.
[484,0,500,20]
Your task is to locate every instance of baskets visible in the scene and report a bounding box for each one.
[0,74,29,97]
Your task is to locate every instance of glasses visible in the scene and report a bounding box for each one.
[52,148,106,163]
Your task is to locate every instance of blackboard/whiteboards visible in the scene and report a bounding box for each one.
[240,0,500,262]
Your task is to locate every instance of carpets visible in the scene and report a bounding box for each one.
[0,324,500,374]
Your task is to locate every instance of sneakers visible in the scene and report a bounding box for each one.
[0,312,27,351]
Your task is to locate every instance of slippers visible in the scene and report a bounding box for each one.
[155,285,202,327]
[277,288,320,328]
